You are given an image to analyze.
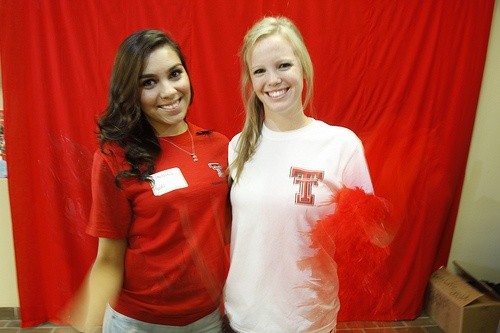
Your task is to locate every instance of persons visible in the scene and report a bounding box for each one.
[222,15,391,333]
[77,28,233,333]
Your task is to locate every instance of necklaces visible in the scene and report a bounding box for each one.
[153,122,200,162]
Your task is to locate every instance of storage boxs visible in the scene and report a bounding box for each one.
[424,261,500,332]
